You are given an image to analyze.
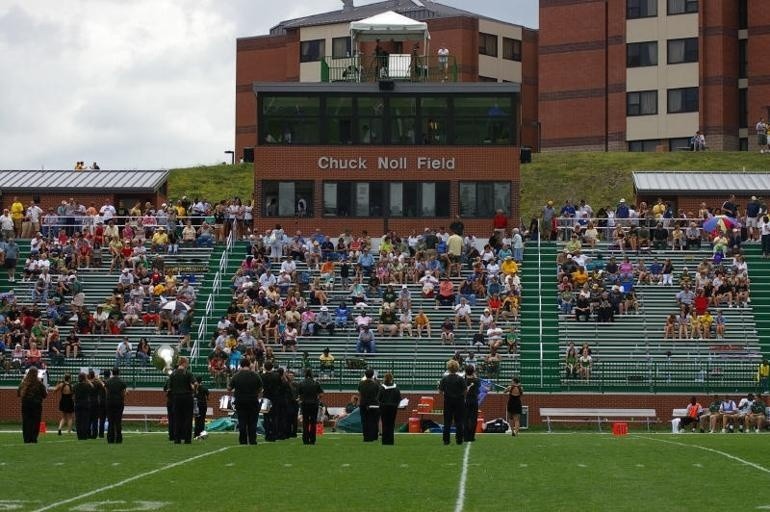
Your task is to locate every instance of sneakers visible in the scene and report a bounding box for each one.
[680,429,760,433]
[59,430,61,435]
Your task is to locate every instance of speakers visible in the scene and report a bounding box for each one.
[521,147,532,164]
[243,147,254,163]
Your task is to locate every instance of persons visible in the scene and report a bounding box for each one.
[89,162,99,169]
[692,131,701,153]
[79,162,85,169]
[208,209,529,448]
[699,130,705,150]
[0,189,254,447]
[755,117,768,153]
[74,162,80,169]
[437,46,449,80]
[766,122,770,154]
[529,188,769,436]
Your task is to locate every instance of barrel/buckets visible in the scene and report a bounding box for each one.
[613,422,628,435]
[39,423,45,432]
[418,404,428,413]
[409,418,421,434]
[309,422,322,435]
[476,418,484,432]
[420,395,433,410]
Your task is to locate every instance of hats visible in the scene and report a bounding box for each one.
[402,285,408,290]
[484,309,490,311]
[122,268,129,272]
[155,227,165,230]
[36,232,42,236]
[320,306,328,311]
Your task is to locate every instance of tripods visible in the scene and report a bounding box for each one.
[404,46,425,82]
[365,42,390,82]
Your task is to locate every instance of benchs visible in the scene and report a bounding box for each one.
[540,407,765,430]
[114,406,360,433]
